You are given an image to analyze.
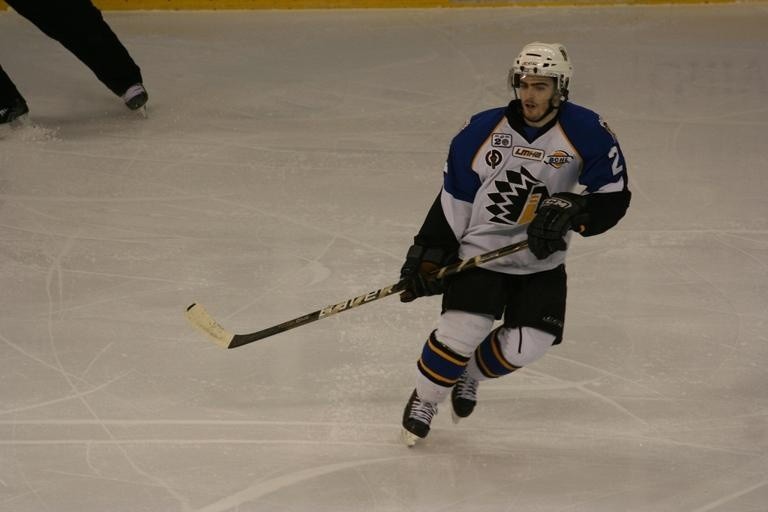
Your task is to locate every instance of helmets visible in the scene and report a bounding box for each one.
[506,41,573,96]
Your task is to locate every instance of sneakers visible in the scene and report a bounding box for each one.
[0,95,29,124]
[451,362,480,419]
[121,83,148,110]
[402,386,437,438]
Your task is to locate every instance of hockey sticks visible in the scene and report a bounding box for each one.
[185,227,573,349]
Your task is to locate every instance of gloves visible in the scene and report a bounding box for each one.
[527,191,595,260]
[400,235,445,302]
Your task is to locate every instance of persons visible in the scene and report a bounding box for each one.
[1,0,151,127]
[399,40,631,440]
